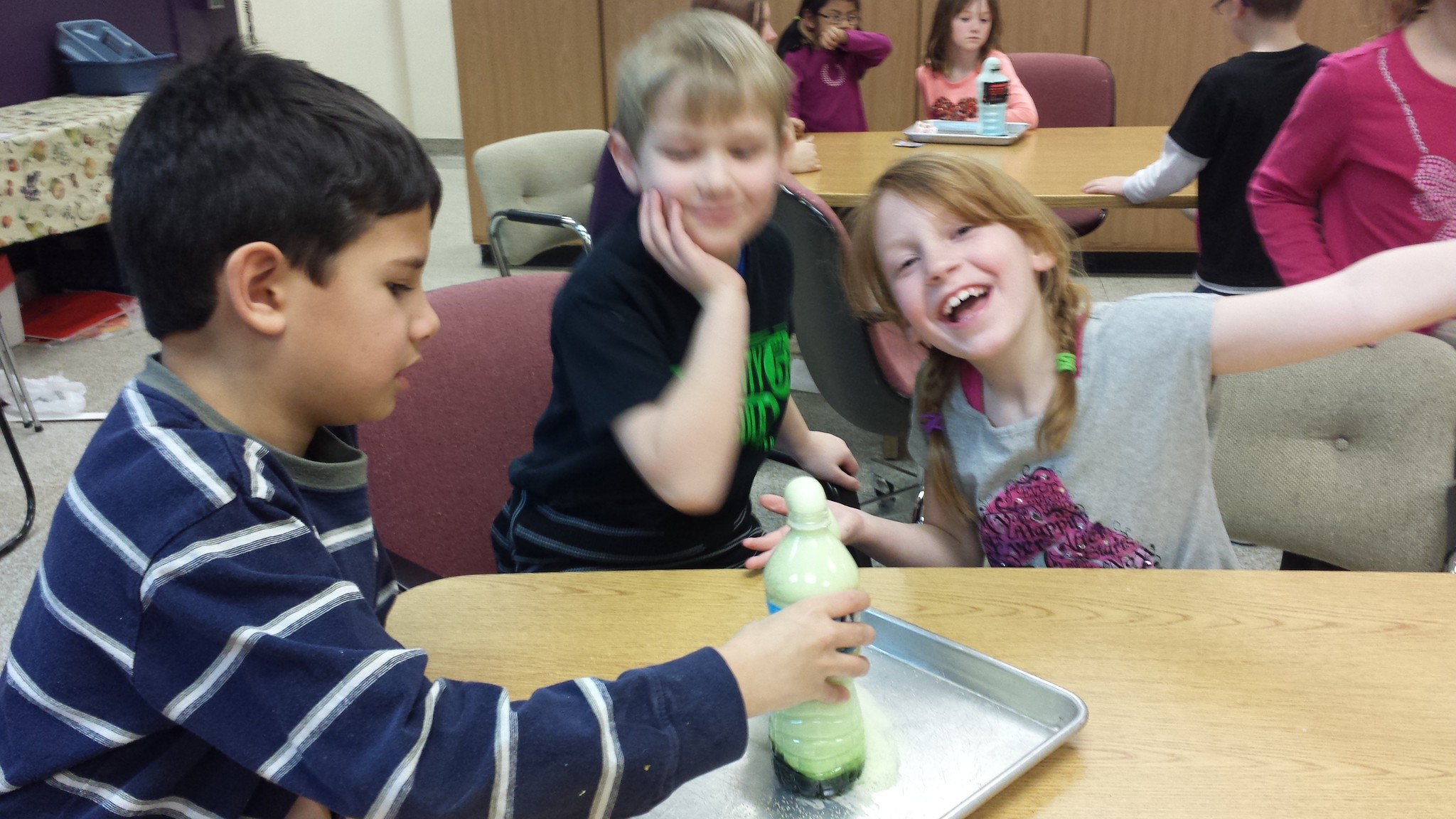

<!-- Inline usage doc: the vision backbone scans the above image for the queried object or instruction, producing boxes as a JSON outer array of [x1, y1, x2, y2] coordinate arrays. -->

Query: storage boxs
[[53, 18, 177, 94]]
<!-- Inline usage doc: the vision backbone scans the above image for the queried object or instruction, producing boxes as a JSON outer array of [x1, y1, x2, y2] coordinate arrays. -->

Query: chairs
[[472, 128, 613, 276], [765, 168, 933, 461], [352, 272, 575, 573], [1001, 52, 1116, 240], [1206, 331, 1456, 572]]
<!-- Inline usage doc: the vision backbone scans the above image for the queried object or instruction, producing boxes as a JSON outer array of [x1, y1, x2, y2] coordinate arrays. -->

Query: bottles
[[763, 505, 868, 796], [975, 59, 1009, 136]]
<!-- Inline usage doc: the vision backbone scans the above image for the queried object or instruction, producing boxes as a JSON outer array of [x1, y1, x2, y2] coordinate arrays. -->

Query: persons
[[774, 2, 893, 138], [1243, 1, 1454, 575], [692, 2, 778, 47], [0, 50, 877, 819], [740, 154, 1455, 574], [1081, 0, 1337, 298], [488, 4, 882, 577], [914, 0, 1040, 134]]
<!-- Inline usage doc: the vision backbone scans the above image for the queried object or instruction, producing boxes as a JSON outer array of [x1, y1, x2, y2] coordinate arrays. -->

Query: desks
[[381, 567, 1456, 819], [0, 94, 156, 249], [787, 125, 1199, 209]]
